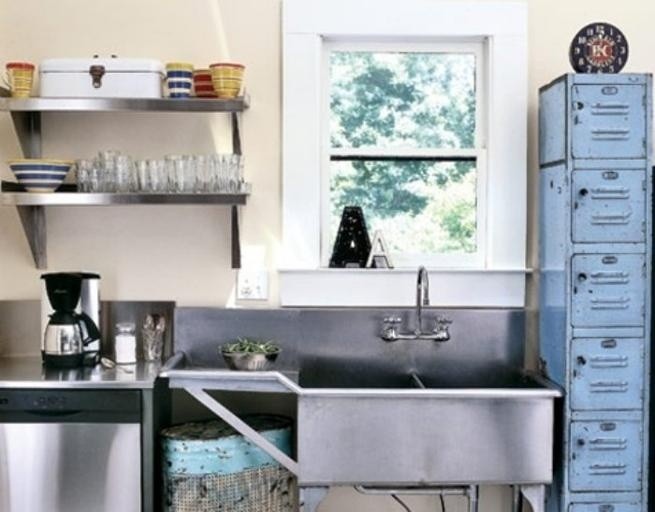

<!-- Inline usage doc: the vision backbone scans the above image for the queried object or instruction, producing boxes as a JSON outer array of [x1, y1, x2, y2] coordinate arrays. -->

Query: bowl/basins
[[6, 158, 74, 193], [219, 347, 282, 370]]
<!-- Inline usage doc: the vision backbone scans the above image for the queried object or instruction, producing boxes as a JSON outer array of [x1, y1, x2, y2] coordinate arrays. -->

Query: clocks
[[569, 23, 629, 74]]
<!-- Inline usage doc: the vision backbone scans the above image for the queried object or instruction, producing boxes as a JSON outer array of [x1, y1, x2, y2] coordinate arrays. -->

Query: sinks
[[413, 372, 566, 486], [297, 370, 425, 487]]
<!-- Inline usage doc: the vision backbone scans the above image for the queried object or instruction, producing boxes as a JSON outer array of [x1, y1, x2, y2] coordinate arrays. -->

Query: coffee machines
[[39, 270, 103, 368]]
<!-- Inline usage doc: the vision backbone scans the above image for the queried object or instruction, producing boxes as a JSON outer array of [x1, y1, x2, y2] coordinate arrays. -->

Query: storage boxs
[[37, 57, 167, 99]]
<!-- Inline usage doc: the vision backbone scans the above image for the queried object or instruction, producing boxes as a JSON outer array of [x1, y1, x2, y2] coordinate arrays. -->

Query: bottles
[[115, 322, 136, 364]]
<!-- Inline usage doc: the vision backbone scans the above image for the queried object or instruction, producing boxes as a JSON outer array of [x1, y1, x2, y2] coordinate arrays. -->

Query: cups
[[164, 62, 245, 99], [142, 326, 163, 363], [76, 151, 246, 196], [2, 62, 35, 96]]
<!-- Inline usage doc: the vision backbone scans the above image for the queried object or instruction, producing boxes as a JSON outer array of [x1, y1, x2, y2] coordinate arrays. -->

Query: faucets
[[417, 267, 429, 332]]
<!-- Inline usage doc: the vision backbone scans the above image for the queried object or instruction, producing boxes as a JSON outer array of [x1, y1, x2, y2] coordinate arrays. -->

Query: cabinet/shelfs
[[539, 73, 653, 168], [539, 158, 653, 512], [0, 87, 252, 270]]
